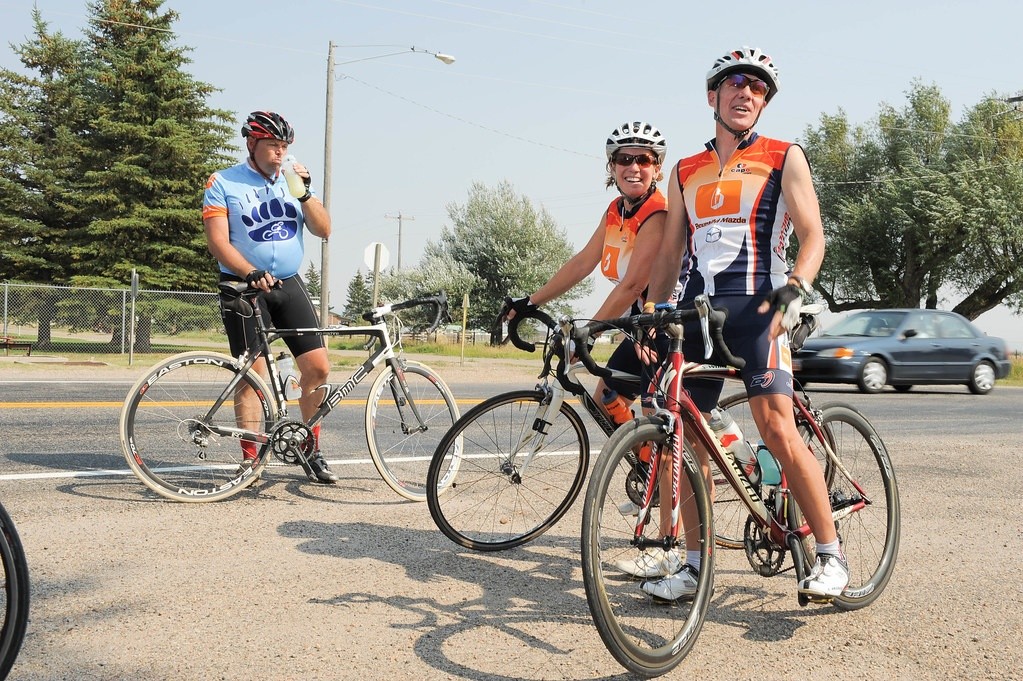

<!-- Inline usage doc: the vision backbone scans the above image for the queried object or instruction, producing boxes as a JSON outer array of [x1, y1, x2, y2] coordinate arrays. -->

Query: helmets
[[241, 111, 294, 144], [707, 46, 781, 105], [606, 122, 668, 166]]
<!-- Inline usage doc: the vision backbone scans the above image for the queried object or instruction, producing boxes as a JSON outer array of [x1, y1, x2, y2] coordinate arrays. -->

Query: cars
[[790, 308, 1013, 394]]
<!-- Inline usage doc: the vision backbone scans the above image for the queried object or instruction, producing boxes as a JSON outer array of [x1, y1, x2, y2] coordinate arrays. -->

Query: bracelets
[[789, 274, 816, 297]]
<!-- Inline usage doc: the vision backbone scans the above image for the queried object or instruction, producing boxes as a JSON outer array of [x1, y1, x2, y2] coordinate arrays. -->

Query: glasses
[[725, 74, 770, 96], [613, 153, 657, 167]]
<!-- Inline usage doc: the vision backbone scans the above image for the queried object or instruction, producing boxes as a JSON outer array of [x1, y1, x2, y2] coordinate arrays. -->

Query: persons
[[633, 48, 850, 602], [499, 120, 688, 578], [203, 112, 338, 487]]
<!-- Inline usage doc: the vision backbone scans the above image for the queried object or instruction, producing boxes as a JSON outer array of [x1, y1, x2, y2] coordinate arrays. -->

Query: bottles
[[756, 437, 782, 485], [639, 440, 653, 463], [709, 408, 760, 485], [601, 387, 634, 425], [275, 351, 302, 401], [282, 154, 306, 199]]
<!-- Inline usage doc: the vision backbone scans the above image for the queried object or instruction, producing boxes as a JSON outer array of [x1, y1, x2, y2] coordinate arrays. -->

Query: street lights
[[319, 39, 457, 348]]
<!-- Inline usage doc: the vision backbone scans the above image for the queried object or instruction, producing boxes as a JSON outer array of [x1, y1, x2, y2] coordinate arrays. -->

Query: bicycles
[[425, 295, 901, 677], [118, 280, 463, 503]]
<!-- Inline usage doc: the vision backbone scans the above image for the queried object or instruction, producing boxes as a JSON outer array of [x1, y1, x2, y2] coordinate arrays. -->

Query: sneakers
[[618, 490, 660, 515], [292, 439, 340, 482], [639, 563, 715, 603], [237, 458, 262, 482], [614, 546, 682, 578], [798, 552, 851, 596]]
